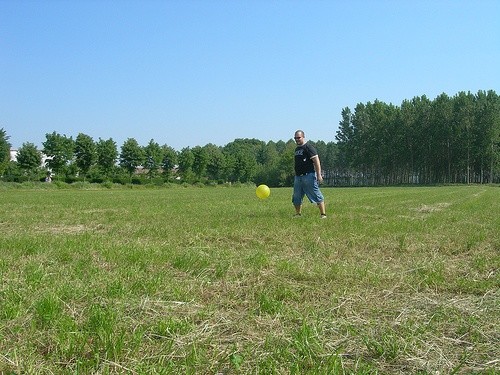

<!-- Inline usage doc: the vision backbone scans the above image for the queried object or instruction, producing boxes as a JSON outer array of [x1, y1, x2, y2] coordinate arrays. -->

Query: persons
[[290, 129, 326, 217]]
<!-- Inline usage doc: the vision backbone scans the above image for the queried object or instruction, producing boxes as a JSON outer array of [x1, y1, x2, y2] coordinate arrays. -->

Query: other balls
[[255, 184, 271, 200]]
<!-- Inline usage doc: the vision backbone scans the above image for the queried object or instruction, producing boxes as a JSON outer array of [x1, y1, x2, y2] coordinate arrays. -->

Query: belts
[[296, 172, 309, 177]]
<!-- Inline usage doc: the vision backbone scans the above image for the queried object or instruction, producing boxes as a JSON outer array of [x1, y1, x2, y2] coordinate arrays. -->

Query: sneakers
[[320, 214, 326, 219], [291, 214, 301, 218]]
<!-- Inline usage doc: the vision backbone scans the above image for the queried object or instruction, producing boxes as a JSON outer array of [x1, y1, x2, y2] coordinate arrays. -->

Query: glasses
[[293, 137, 301, 139]]
